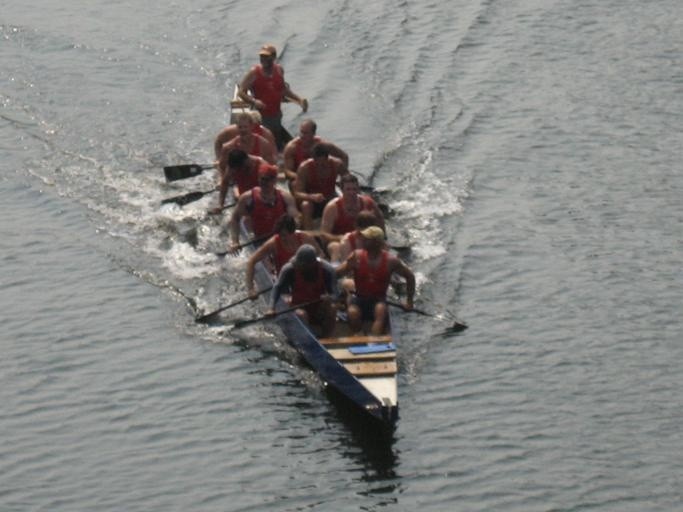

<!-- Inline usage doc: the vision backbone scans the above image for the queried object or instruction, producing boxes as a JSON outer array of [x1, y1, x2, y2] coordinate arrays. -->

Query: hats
[[257, 44, 276, 55], [361, 226, 384, 240], [259, 165, 278, 177], [289, 244, 316, 267], [308, 144, 329, 155]]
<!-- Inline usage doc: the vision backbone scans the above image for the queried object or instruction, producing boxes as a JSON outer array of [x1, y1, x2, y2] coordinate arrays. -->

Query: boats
[[221, 83, 401, 427]]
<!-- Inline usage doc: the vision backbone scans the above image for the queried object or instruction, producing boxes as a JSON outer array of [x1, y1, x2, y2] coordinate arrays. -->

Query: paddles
[[377, 202, 393, 218], [164, 164, 215, 180], [350, 289, 468, 330], [235, 297, 319, 327], [162, 188, 217, 205], [194, 286, 272, 321], [390, 245, 407, 253], [334, 180, 373, 193]]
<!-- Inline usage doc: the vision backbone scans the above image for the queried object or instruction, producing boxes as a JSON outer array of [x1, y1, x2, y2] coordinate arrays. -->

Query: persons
[[215, 110, 415, 334], [238, 46, 308, 148]]
